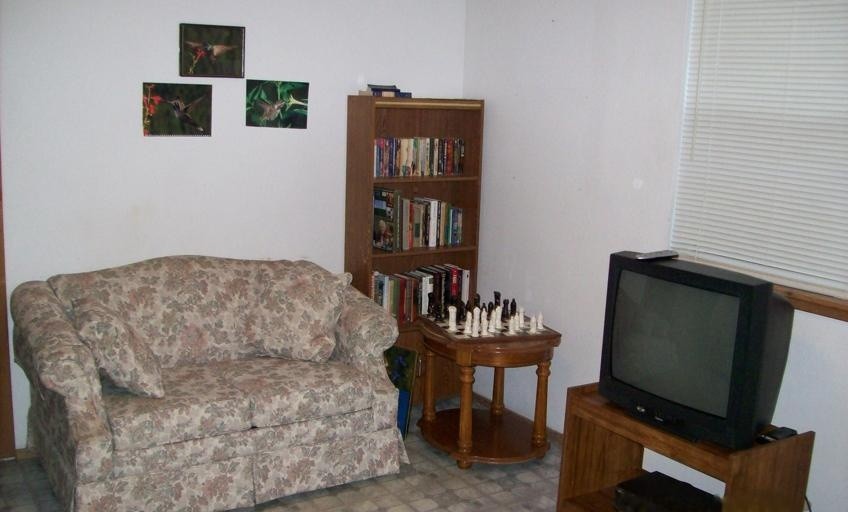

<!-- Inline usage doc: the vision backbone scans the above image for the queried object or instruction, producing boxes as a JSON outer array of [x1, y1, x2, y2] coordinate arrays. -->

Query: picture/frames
[[180, 22, 245, 78]]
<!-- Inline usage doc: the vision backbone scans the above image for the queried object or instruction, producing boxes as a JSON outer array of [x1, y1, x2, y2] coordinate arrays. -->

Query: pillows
[[71, 268, 352, 398]]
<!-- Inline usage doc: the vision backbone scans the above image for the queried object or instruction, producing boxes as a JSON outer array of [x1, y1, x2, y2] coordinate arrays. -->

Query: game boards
[[421, 308, 557, 340]]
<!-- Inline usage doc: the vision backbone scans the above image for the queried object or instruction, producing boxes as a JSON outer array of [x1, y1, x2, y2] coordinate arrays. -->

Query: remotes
[[634, 249, 679, 263]]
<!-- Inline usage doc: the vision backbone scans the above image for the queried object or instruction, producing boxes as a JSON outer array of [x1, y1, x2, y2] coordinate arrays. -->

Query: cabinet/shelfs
[[555, 381, 814, 512], [344, 94, 485, 405]]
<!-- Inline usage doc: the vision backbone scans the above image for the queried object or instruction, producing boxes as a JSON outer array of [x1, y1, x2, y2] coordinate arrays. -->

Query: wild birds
[[253, 98, 286, 122], [165, 94, 207, 134], [185, 37, 238, 60]]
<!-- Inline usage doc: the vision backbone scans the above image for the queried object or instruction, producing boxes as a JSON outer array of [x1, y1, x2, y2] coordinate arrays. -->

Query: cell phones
[[759, 426, 796, 444]]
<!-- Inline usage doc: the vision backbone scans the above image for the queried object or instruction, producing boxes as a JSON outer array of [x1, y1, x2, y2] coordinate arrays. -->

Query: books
[[373, 134, 473, 326], [367, 83, 399, 91], [358, 90, 412, 99]]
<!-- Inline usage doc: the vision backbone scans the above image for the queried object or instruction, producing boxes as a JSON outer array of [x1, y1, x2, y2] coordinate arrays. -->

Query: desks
[[420, 318, 561, 468]]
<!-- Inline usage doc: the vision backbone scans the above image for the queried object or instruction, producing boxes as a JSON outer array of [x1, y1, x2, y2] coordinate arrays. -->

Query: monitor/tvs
[[597, 250, 794, 453]]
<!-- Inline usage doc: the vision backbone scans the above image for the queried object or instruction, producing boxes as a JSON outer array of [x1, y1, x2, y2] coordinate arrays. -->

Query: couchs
[[10, 256, 412, 512]]
